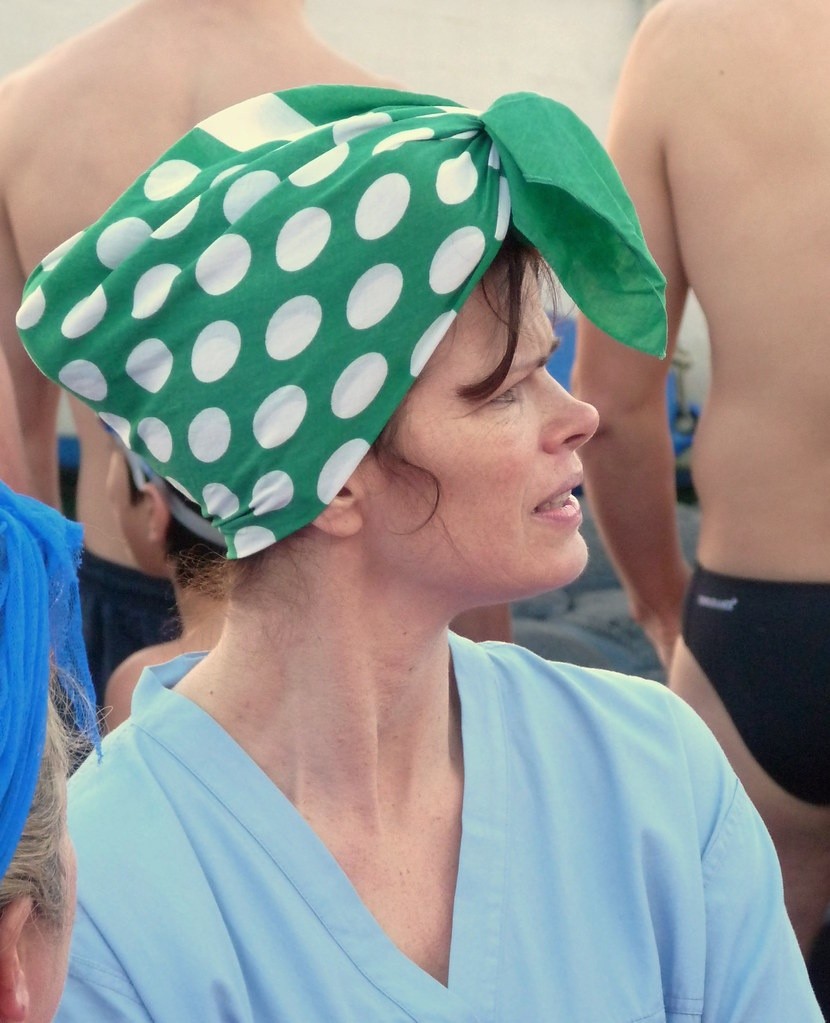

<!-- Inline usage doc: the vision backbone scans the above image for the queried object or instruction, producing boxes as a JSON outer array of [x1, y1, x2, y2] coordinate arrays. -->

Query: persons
[[49, 86, 824, 1023], [568, 0, 830, 964], [0, 478, 111, 1023], [0, 0, 508, 736]]
